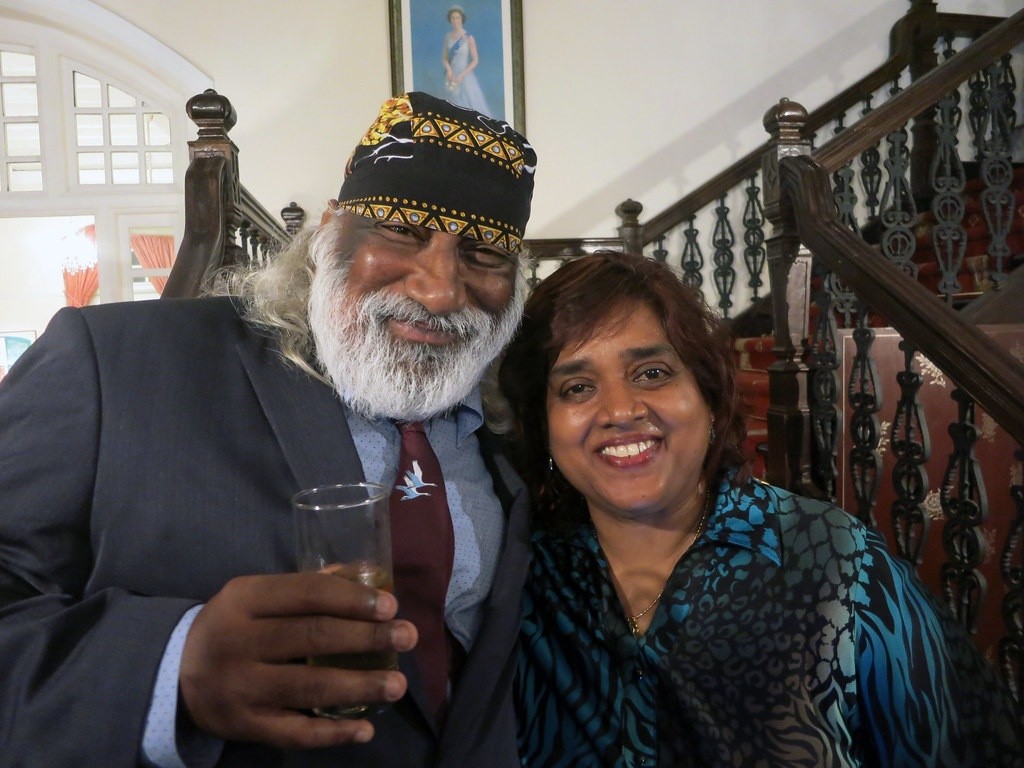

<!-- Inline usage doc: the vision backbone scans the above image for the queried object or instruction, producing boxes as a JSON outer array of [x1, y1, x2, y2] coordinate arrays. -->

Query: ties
[[388, 420, 455, 740]]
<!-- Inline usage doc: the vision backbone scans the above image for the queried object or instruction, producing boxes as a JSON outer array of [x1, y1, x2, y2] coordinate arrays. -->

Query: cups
[[288, 482, 401, 721]]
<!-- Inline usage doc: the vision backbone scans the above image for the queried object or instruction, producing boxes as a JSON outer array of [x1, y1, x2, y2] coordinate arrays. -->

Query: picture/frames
[[389, 1, 526, 141]]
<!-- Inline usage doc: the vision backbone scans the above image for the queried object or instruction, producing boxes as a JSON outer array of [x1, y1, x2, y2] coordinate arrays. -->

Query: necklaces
[[626, 490, 710, 635]]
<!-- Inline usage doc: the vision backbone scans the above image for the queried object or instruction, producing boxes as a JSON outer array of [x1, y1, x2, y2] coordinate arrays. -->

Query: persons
[[439, 7, 488, 112], [499, 250, 1024, 768], [0, 91, 537, 768]]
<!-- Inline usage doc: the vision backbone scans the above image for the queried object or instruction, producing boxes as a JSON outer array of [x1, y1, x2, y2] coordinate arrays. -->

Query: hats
[[338, 92, 538, 255]]
